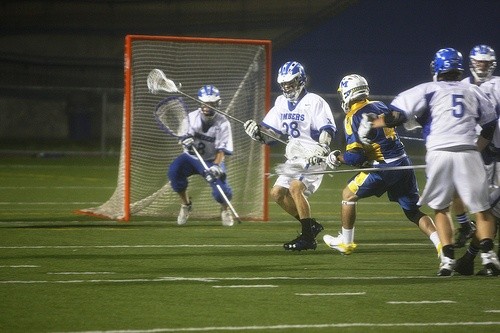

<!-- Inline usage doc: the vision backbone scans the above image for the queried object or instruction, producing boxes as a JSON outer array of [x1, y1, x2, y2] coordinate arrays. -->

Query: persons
[[323, 74, 442, 258], [167, 85, 235, 226], [244, 61, 336, 250], [357, 49, 500, 277], [455, 45, 500, 248]]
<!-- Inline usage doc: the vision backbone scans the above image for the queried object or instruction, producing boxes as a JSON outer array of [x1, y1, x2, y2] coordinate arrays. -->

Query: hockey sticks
[[152, 95, 242, 224], [274, 163, 426, 177], [146, 68, 288, 146], [286, 139, 346, 164]]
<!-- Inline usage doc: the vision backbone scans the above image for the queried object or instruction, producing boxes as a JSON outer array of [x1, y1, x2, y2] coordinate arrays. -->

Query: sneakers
[[453, 256, 474, 275], [221, 207, 234, 226], [475, 255, 500, 276], [481, 249, 500, 276], [177, 201, 192, 225], [454, 221, 477, 248], [322, 231, 357, 256], [283, 236, 317, 252], [300, 222, 324, 239], [437, 256, 455, 276]]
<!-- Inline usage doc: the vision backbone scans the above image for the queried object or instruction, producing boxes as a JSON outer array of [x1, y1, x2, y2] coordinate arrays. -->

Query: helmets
[[429, 48, 464, 82], [337, 74, 369, 115], [276, 61, 307, 102], [469, 45, 497, 83], [198, 85, 222, 120]]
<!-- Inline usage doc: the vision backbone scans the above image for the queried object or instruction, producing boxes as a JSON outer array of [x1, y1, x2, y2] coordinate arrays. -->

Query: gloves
[[203, 165, 225, 183], [243, 120, 265, 144], [326, 150, 341, 170], [304, 143, 331, 166], [357, 113, 376, 146], [179, 134, 195, 150]]
